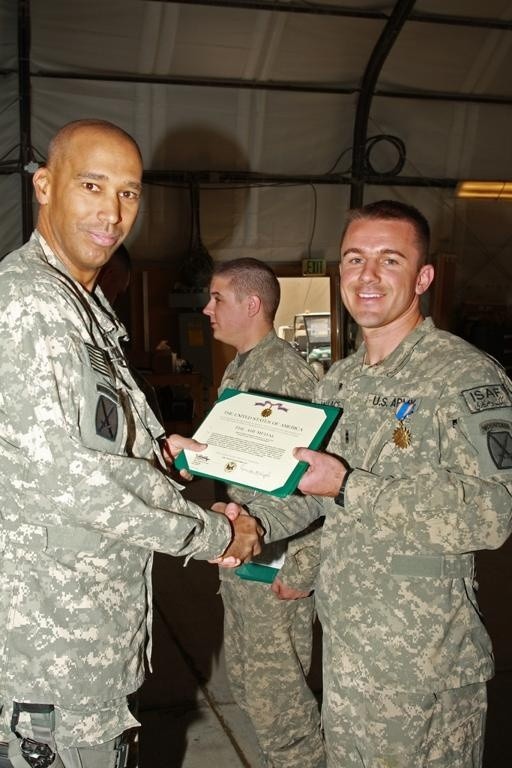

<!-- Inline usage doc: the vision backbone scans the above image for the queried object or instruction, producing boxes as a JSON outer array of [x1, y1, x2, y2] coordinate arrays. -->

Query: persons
[[205, 196, 511, 767], [195, 255, 328, 768], [0, 116, 268, 768]]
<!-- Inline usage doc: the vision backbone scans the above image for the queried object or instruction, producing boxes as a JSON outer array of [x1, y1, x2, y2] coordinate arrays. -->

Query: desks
[[131, 369, 204, 437]]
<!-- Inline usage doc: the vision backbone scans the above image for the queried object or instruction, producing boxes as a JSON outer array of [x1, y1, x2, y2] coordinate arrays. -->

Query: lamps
[[453, 180, 511, 200]]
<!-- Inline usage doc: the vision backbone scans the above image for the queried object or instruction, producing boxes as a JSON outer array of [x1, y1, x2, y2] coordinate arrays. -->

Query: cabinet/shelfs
[[293, 312, 332, 364]]
